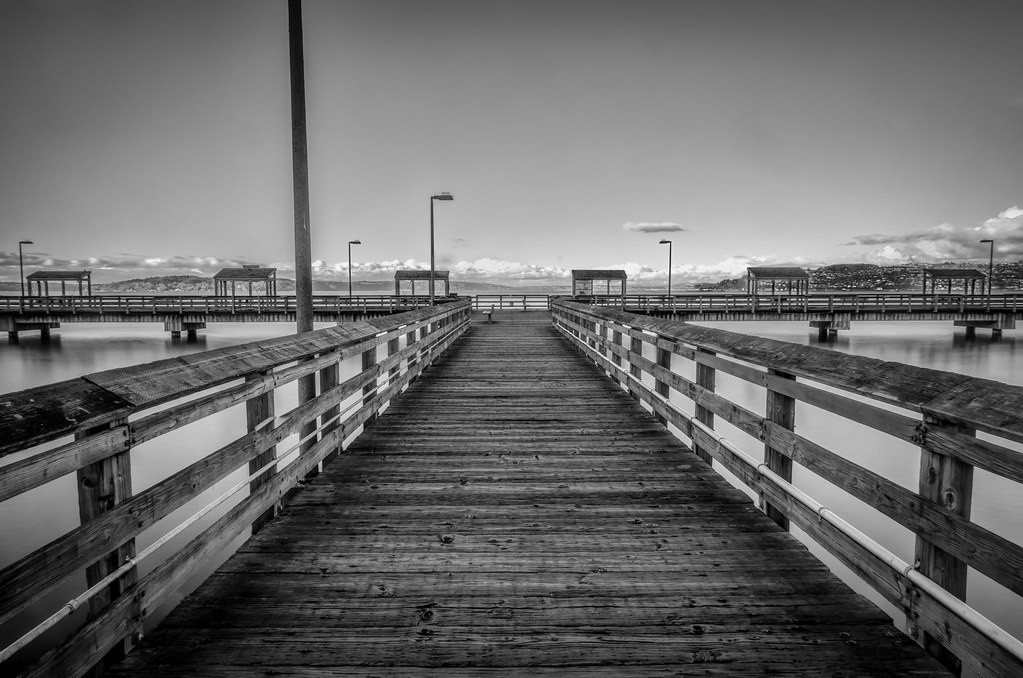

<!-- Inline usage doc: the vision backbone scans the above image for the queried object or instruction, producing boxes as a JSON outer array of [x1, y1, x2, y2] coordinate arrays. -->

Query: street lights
[[19, 241, 33, 311], [348, 240, 361, 308], [659, 240, 672, 308], [429, 194, 454, 307], [980, 239, 995, 312]]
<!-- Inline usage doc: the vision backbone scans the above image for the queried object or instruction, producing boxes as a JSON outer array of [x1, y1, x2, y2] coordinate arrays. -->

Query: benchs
[[483, 304, 494, 324]]
